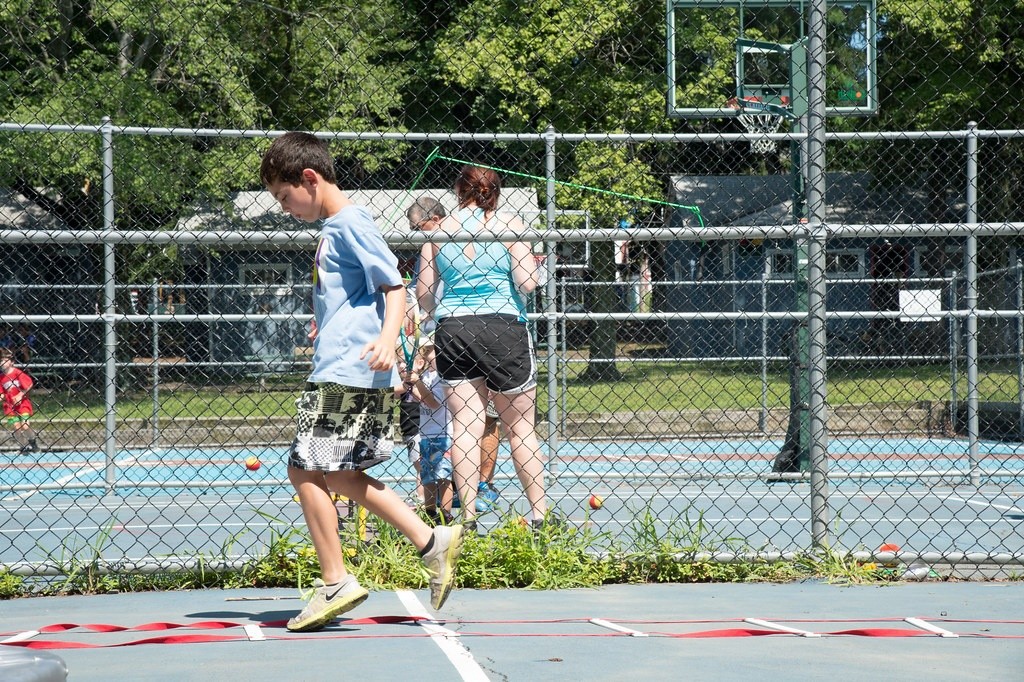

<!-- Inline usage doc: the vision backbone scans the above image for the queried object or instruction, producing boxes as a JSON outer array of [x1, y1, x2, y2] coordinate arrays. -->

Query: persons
[[416, 162, 568, 543], [259, 132, 465, 632], [402, 329, 454, 529], [0, 349, 40, 457], [406, 197, 502, 514], [0, 324, 39, 368], [394, 341, 431, 507]]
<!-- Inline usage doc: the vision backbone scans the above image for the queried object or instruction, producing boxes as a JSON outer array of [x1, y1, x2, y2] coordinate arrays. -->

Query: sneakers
[[417, 525, 464, 610], [19, 447, 29, 456], [29, 439, 41, 453], [439, 511, 457, 525], [403, 495, 427, 511], [436, 493, 462, 508], [286, 576, 370, 632], [474, 484, 501, 512], [461, 519, 479, 540], [532, 516, 570, 541]]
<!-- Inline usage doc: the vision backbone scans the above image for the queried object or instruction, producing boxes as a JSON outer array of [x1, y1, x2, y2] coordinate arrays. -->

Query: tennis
[[587, 495, 603, 508], [245, 456, 260, 470]]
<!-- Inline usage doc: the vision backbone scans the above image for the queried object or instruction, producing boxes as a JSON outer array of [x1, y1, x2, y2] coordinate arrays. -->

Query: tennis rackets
[[400, 287, 422, 382]]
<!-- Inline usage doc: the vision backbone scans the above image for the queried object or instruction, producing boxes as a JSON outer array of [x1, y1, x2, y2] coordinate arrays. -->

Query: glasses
[[410, 215, 431, 232]]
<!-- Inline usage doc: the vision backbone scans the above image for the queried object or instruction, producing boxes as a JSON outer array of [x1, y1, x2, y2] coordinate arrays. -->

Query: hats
[[418, 331, 435, 346]]
